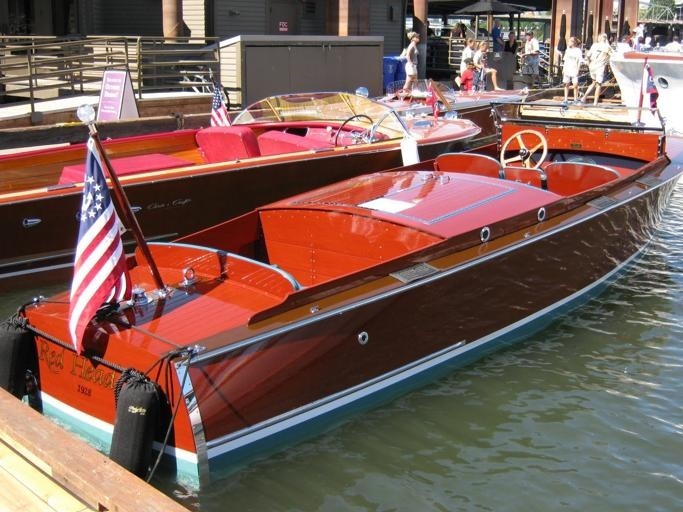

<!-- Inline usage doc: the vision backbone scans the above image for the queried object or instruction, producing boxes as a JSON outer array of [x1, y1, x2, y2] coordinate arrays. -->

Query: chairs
[[258, 131, 326, 155], [432, 153, 504, 182], [195, 127, 258, 161], [542, 160, 620, 196]]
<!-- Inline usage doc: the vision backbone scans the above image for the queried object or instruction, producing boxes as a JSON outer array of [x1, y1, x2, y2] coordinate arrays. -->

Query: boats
[[0, 87, 487, 294], [606, 49, 682, 137], [0, 57, 682, 500], [316, 85, 534, 157]]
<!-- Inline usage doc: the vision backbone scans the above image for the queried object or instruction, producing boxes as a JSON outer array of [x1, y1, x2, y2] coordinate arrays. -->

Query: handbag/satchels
[[400, 49, 410, 62]]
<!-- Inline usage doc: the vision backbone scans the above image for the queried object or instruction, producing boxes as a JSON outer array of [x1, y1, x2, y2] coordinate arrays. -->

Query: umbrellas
[[453, 1, 521, 39], [557, 11, 566, 73]]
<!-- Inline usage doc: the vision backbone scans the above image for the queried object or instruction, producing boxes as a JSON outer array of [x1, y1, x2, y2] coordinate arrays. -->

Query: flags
[[642, 62, 657, 115], [66, 134, 132, 359], [209, 86, 231, 127]]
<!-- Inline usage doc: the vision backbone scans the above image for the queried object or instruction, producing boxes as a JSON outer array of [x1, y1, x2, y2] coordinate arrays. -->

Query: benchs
[[130, 239, 298, 300]]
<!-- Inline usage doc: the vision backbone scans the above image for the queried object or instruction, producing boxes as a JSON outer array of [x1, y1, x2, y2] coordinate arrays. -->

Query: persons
[[459, 37, 476, 76], [397, 31, 421, 93], [491, 19, 504, 51], [580, 32, 614, 106], [472, 40, 504, 91], [521, 30, 540, 86], [562, 37, 583, 104], [607, 19, 682, 55], [503, 32, 519, 72]]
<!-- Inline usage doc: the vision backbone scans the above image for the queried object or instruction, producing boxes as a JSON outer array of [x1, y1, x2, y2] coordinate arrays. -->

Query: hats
[[408, 32, 420, 39], [526, 31, 534, 35]]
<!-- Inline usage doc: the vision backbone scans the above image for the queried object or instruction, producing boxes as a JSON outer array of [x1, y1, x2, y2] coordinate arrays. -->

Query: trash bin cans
[[383, 55, 406, 90]]
